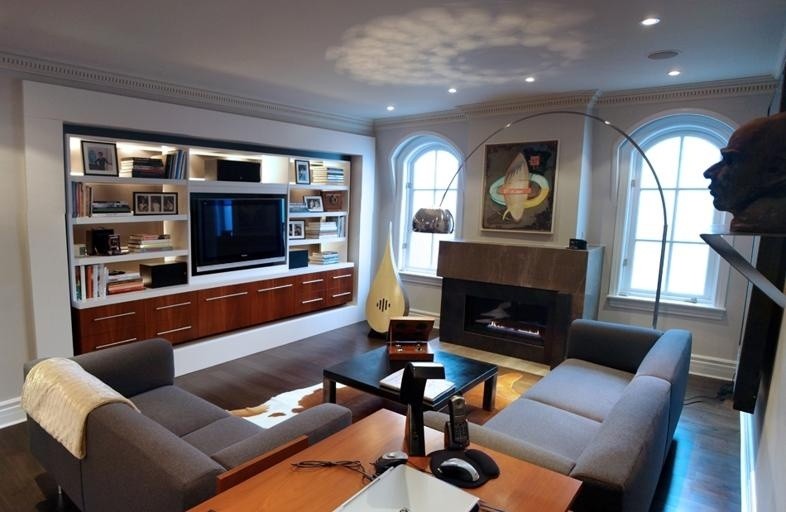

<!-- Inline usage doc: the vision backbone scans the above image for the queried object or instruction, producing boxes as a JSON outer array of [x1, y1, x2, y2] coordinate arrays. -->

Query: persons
[[700, 110, 786, 236]]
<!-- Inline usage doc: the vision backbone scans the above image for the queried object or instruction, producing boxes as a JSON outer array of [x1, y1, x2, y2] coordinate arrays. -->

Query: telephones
[[444, 396, 469, 450]]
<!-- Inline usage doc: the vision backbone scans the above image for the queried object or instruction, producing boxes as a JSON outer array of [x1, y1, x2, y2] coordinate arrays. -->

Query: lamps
[[410, 109, 669, 331]]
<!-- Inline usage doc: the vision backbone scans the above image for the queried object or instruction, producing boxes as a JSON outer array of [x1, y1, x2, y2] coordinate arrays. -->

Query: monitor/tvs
[[190, 193, 287, 276]]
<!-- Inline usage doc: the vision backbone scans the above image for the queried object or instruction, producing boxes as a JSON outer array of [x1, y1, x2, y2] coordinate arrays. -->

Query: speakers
[[140, 261, 187, 287], [207, 159, 260, 182], [289, 247, 308, 269]]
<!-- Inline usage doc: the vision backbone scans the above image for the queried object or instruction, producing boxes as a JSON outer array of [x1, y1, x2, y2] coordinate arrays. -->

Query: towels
[[18, 356, 142, 461]]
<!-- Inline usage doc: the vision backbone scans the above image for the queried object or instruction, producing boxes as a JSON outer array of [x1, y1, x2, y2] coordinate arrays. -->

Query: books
[[379, 385, 457, 407], [379, 365, 456, 402], [71, 148, 185, 301], [288, 160, 344, 268]]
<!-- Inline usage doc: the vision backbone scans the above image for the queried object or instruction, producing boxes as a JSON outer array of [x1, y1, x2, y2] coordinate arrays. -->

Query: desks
[[184, 408, 585, 512]]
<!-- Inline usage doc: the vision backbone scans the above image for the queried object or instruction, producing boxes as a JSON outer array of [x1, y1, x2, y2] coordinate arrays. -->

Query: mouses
[[437, 458, 481, 483]]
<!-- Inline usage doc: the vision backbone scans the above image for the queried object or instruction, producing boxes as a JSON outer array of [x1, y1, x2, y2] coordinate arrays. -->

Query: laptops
[[331, 464, 480, 512]]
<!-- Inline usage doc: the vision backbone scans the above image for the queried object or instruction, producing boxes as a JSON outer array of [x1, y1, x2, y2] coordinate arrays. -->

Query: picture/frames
[[293, 159, 310, 185], [131, 191, 178, 216], [80, 139, 119, 177], [302, 195, 325, 213], [64, 132, 189, 310], [288, 219, 305, 240], [477, 135, 561, 236], [729, 56, 785, 416], [319, 190, 343, 211]]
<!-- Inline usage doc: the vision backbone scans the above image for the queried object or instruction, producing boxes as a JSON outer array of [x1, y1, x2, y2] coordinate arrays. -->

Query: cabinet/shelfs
[[287, 154, 350, 276], [295, 270, 325, 316], [196, 282, 253, 338], [69, 300, 144, 355], [144, 289, 196, 349], [326, 266, 354, 310], [252, 276, 295, 326]]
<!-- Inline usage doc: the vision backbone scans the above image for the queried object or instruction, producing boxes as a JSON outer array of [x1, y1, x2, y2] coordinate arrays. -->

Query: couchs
[[421, 318, 693, 512], [18, 337, 353, 512]]
